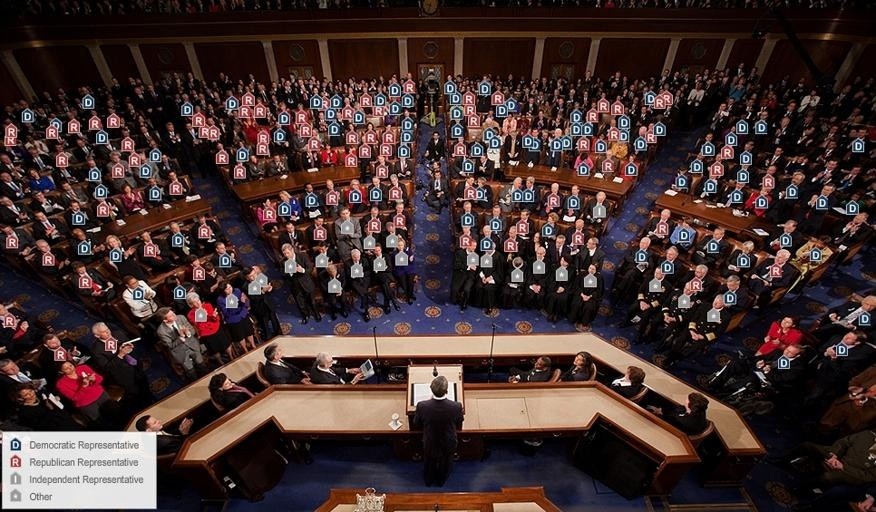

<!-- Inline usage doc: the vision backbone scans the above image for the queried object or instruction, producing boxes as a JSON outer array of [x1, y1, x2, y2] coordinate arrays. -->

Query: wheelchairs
[[702, 349, 776, 419]]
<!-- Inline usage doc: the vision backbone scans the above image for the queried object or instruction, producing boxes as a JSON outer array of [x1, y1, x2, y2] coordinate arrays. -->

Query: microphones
[[433, 360, 439, 376], [368, 326, 376, 331], [492, 323, 503, 329]]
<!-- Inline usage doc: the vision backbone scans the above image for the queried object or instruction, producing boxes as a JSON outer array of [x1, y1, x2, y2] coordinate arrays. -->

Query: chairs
[[256, 91, 417, 303], [689, 420, 715, 450], [254, 362, 273, 388], [209, 393, 227, 415], [646, 137, 876, 397], [546, 369, 563, 383], [443, 71, 611, 328], [1, 105, 254, 428], [629, 387, 649, 404], [587, 362, 597, 381]]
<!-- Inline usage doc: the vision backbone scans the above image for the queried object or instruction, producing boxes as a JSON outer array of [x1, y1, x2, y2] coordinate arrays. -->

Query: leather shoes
[[451, 295, 593, 332], [604, 313, 685, 367]]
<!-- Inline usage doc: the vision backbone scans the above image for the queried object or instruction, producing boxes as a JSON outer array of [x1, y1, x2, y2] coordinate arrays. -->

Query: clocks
[[418, 0, 442, 18]]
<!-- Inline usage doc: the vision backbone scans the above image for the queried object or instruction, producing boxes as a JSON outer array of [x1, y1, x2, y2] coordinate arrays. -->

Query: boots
[[294, 281, 417, 324]]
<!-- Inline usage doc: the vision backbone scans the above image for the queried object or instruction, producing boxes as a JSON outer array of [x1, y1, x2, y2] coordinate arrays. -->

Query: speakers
[[572, 429, 656, 500], [215, 426, 290, 504]]
